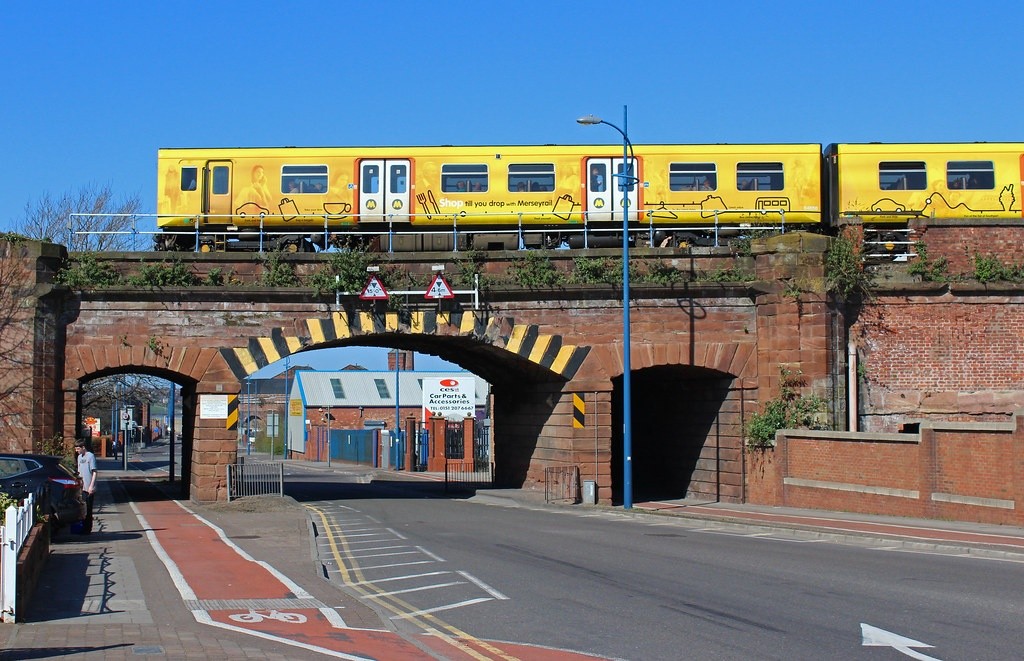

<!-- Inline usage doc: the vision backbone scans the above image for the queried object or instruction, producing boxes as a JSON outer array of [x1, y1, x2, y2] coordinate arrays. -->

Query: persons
[[287, 180, 324, 194], [591, 168, 602, 192], [456, 180, 548, 192], [74, 439, 97, 535]]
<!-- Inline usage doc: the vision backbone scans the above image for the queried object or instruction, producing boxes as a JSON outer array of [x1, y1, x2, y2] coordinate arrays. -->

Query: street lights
[[576, 104, 635, 512]]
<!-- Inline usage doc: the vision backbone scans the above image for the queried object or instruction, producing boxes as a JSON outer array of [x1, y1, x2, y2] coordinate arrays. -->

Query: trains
[[159, 135, 1024, 253]]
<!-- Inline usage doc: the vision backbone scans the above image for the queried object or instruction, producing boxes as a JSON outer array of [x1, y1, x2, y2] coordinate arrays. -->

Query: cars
[[0, 451, 88, 537]]
